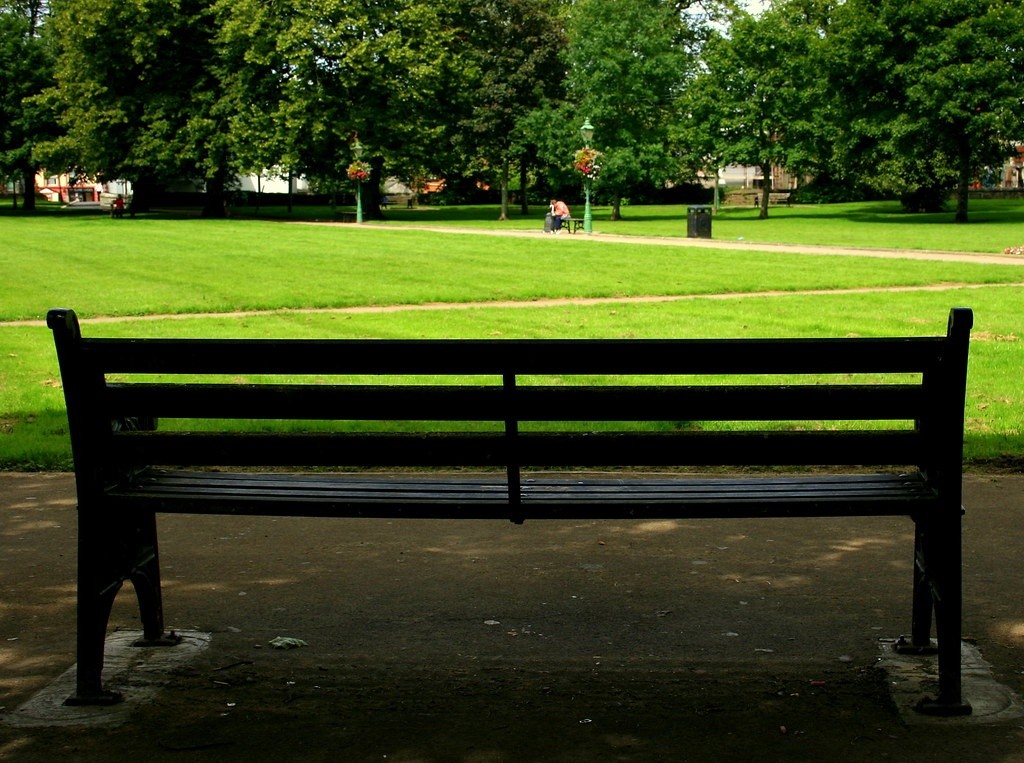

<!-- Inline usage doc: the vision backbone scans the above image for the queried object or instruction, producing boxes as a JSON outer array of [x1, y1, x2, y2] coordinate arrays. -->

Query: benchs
[[46, 308, 977, 720], [110, 202, 151, 219], [757, 193, 793, 209], [553, 219, 584, 234]]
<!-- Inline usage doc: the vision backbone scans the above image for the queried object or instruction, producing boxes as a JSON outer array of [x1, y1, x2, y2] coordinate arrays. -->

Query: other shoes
[[556, 229, 561, 234], [551, 229, 556, 234]]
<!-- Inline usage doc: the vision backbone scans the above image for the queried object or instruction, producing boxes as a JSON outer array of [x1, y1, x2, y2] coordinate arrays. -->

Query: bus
[[38, 185, 98, 205]]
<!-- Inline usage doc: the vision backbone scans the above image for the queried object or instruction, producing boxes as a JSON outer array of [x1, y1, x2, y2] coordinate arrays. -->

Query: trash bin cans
[[687, 205, 712, 238]]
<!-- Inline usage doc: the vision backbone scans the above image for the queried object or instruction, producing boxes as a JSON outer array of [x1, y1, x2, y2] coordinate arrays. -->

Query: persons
[[407, 187, 413, 209], [381, 195, 388, 209], [550, 200, 569, 234], [111, 194, 123, 219]]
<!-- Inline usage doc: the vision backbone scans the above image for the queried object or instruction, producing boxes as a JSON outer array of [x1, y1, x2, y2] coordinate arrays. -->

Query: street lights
[[579, 116, 594, 234]]
